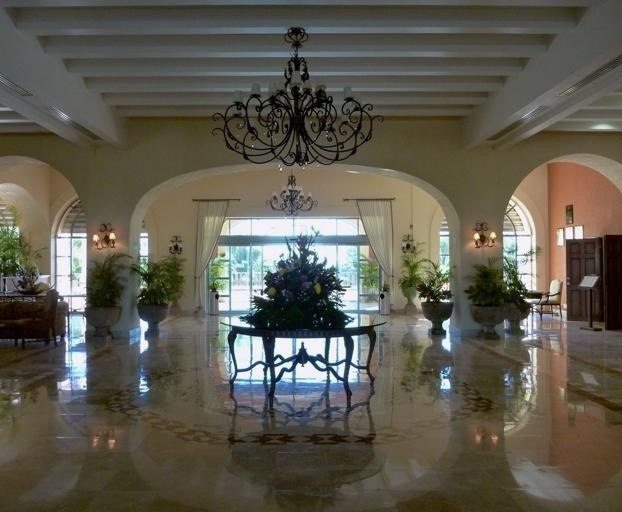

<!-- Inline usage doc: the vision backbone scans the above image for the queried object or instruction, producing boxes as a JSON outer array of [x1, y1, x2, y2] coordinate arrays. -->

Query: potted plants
[[418, 337, 453, 404], [497, 252, 533, 335], [462, 256, 506, 339], [394, 233, 438, 316], [84, 251, 133, 345], [159, 255, 184, 315], [416, 257, 458, 336], [206, 261, 226, 292], [139, 339, 184, 392], [397, 327, 426, 394], [127, 252, 187, 337]]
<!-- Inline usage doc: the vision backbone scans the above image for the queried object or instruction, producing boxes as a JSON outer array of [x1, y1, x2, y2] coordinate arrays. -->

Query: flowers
[[238, 225, 355, 331], [229, 443, 375, 511]]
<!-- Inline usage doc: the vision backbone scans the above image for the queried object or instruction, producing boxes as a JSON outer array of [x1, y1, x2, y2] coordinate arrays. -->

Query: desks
[[522, 292, 550, 321], [221, 311, 388, 417], [222, 423, 385, 488], [0, 292, 47, 303]]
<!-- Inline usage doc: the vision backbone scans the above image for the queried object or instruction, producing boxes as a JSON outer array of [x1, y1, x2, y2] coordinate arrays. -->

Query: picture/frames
[[556, 203, 585, 247]]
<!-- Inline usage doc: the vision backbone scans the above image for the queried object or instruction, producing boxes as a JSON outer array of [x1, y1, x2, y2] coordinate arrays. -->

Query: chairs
[[532, 279, 564, 321]]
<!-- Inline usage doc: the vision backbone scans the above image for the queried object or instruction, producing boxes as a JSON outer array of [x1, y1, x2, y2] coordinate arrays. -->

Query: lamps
[[208, 37, 385, 174], [473, 221, 497, 249], [264, 168, 318, 216], [168, 234, 183, 254], [92, 223, 118, 251]]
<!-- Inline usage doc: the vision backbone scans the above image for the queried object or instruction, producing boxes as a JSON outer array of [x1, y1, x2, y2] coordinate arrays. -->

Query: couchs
[[0, 289, 60, 349]]
